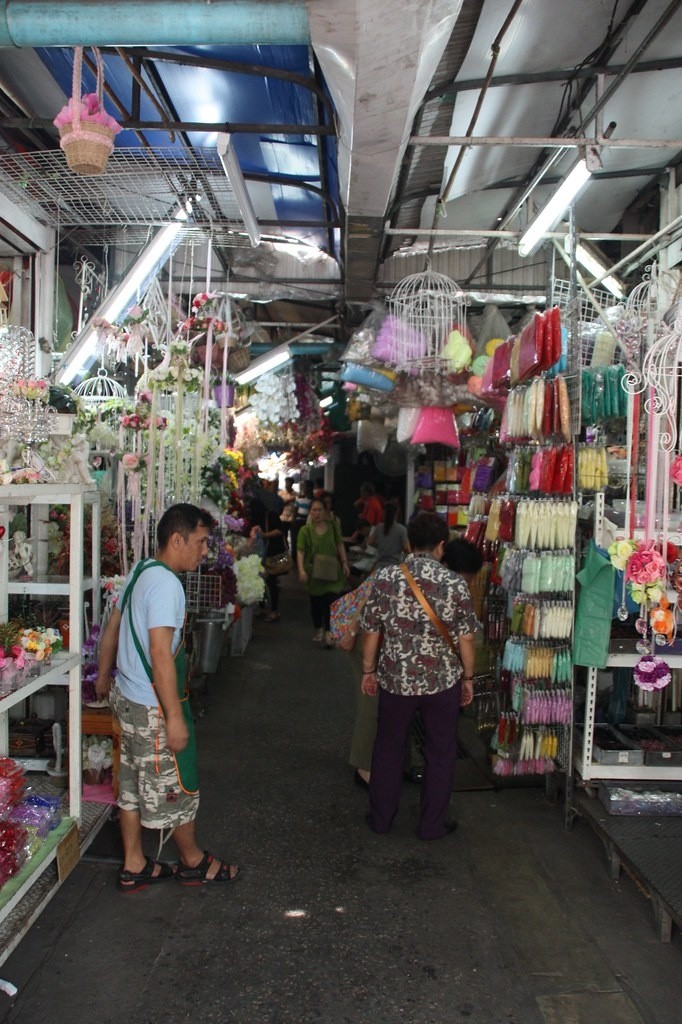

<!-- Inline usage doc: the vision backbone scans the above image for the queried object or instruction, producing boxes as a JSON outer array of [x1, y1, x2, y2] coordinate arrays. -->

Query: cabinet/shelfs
[[422, 278, 682, 831], [0, 481, 141, 966]]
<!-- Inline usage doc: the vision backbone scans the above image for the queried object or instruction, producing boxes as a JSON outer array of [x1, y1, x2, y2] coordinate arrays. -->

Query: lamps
[[518, 147, 603, 258], [563, 222, 628, 302], [217, 131, 261, 247], [56, 185, 204, 388], [235, 347, 294, 386]]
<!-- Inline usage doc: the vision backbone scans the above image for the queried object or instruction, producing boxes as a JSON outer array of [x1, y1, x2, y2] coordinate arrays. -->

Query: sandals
[[116, 856, 178, 894], [175, 850, 240, 885]]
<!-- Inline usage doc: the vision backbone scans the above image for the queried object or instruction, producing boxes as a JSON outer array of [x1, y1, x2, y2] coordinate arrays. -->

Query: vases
[[194, 343, 225, 369], [228, 347, 251, 373], [214, 385, 234, 408], [215, 333, 238, 349]]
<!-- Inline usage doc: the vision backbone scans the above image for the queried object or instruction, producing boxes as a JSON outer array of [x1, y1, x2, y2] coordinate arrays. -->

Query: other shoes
[[326, 638, 335, 647], [355, 768, 369, 790], [255, 607, 265, 616], [264, 611, 280, 622], [313, 636, 324, 643], [448, 820, 457, 833]]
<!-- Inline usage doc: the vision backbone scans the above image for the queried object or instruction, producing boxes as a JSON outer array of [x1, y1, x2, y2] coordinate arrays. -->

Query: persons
[[95, 503, 242, 895], [367, 501, 411, 568], [291, 479, 315, 569], [351, 481, 385, 540], [439, 537, 484, 591], [275, 476, 298, 540], [306, 489, 341, 536], [295, 496, 350, 645], [249, 502, 290, 621], [356, 510, 476, 841]]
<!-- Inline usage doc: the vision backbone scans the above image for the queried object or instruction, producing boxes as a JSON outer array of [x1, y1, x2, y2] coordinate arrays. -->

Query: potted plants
[[35, 387, 78, 437]]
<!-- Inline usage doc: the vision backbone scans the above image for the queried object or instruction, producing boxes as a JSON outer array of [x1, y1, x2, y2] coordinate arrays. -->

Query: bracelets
[[361, 668, 376, 675], [342, 559, 348, 562], [461, 675, 474, 680]]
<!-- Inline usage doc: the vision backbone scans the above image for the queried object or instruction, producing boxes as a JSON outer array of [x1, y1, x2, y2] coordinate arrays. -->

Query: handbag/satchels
[[329, 566, 381, 654], [314, 554, 338, 582], [266, 551, 293, 577]]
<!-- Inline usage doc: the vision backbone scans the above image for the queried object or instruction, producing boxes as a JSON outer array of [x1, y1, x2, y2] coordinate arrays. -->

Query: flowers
[[606, 538, 682, 692], [1, 293, 267, 886], [54, 94, 123, 135]]
